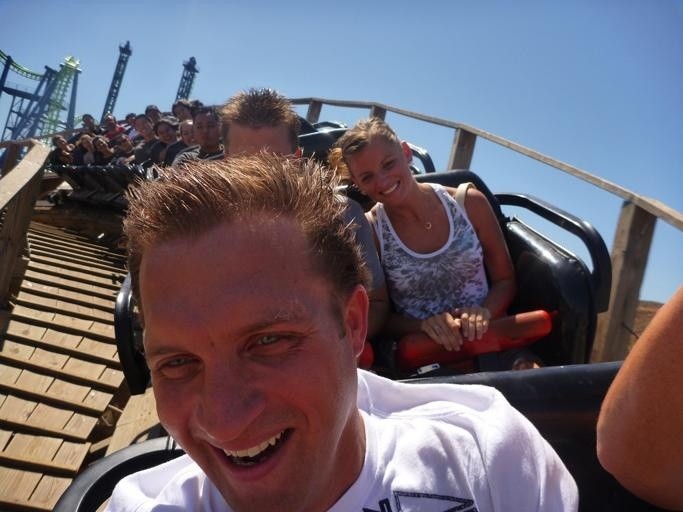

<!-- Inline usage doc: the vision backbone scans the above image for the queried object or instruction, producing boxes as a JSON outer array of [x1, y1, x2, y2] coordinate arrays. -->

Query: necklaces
[[389, 185, 433, 230]]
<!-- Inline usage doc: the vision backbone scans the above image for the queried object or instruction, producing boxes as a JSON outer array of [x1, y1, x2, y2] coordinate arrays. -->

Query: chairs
[[334, 167, 612, 372], [49, 131, 80, 166], [203, 128, 352, 170], [52, 357, 634, 511]]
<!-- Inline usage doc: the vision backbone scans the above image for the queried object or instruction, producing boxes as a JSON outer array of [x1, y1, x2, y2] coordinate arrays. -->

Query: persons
[[132, 113, 159, 161], [53, 135, 78, 165], [81, 135, 97, 164], [218, 87, 388, 338], [124, 113, 141, 139], [101, 150, 580, 512], [146, 105, 162, 124], [172, 107, 225, 167], [174, 119, 200, 160], [92, 136, 120, 165], [104, 113, 126, 139], [113, 133, 136, 163], [339, 118, 540, 380], [81, 112, 103, 135], [172, 97, 192, 120], [596, 284, 681, 511], [155, 120, 179, 162]]
